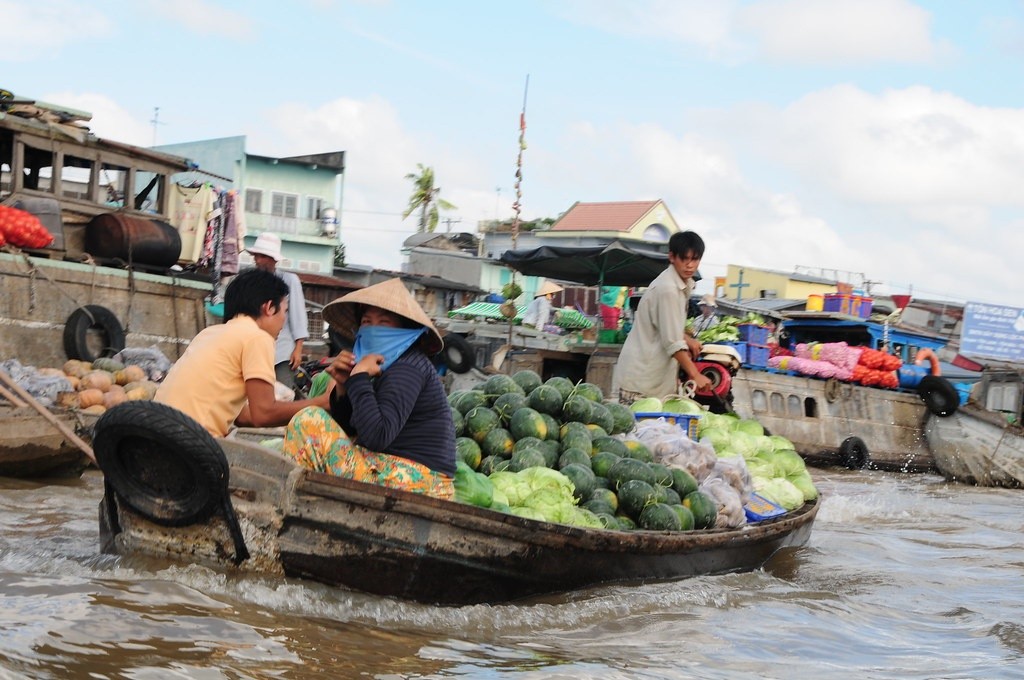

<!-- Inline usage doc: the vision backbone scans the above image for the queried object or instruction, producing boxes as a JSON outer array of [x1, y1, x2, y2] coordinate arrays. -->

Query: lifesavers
[[914, 347, 941, 376]]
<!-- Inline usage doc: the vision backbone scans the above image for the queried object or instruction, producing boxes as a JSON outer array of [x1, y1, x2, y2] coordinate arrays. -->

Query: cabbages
[[630, 394, 817, 508]]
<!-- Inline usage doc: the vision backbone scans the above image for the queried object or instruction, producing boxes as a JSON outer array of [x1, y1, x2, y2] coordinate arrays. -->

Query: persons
[[689, 294, 719, 334], [321, 276, 456, 479], [520, 281, 564, 338], [153, 267, 337, 442], [617, 231, 712, 407], [241, 233, 310, 404]]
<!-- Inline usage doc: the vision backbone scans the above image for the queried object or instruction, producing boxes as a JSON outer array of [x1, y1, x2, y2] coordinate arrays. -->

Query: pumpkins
[[41, 357, 157, 414]]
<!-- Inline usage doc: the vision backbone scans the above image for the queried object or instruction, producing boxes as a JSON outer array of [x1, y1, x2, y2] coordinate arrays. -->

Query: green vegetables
[[686, 312, 766, 344]]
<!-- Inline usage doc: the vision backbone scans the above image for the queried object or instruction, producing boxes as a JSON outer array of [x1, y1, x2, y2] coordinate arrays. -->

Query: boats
[[23, 313, 1024, 595]]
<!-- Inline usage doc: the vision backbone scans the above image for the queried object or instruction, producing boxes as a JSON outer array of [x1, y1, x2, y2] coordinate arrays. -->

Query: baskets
[[741, 492, 787, 523], [584, 327, 627, 344], [636, 413, 700, 444], [717, 325, 771, 363]]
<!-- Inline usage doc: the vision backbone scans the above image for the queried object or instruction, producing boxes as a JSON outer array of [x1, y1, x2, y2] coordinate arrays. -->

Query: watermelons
[[445, 369, 717, 533]]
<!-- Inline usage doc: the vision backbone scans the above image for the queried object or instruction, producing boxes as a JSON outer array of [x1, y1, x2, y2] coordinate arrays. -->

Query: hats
[[697, 295, 718, 308], [245, 233, 285, 262], [532, 281, 565, 299], [322, 276, 444, 357]]
